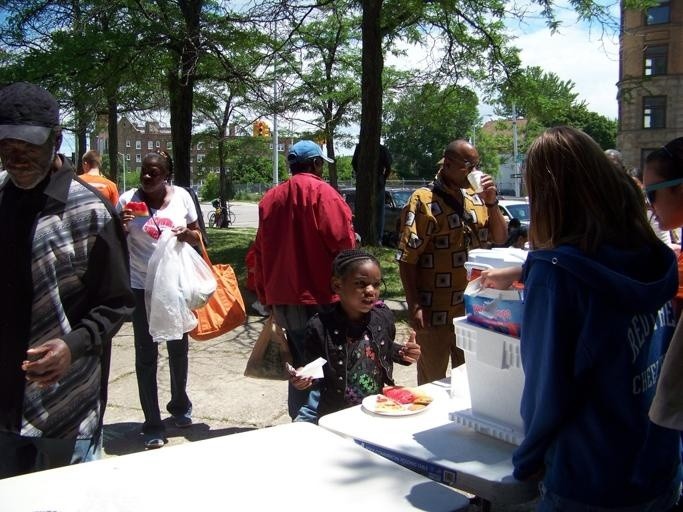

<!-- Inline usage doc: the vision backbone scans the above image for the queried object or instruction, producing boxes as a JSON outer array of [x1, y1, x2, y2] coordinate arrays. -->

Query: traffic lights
[[256, 121, 265, 139], [263, 122, 272, 139]]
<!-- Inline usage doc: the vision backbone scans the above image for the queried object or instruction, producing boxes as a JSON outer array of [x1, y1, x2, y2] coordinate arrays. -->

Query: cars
[[496, 199, 532, 238]]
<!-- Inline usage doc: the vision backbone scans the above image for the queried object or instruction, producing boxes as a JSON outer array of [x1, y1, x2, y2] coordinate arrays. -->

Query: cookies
[[376, 386, 431, 412]]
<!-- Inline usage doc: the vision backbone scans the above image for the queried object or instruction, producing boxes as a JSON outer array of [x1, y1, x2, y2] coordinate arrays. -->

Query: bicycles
[[207, 196, 236, 228]]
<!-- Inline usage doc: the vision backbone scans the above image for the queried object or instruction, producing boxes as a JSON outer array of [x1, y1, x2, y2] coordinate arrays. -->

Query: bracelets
[[484, 199, 498, 209]]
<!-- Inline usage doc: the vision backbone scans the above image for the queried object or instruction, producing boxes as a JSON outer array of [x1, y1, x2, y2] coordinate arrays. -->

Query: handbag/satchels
[[188, 264, 246, 341]]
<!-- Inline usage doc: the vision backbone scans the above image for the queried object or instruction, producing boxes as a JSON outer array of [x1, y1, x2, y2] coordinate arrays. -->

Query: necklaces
[[143, 189, 164, 210]]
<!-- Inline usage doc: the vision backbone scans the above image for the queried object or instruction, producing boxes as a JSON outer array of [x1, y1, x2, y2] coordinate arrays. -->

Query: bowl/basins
[[646, 210, 671, 251]]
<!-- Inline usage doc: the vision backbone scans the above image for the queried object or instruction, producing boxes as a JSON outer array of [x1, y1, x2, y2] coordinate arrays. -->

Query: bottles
[[359, 392, 435, 416]]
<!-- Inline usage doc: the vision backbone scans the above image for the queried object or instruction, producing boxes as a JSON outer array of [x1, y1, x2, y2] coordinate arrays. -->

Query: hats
[[0, 82, 59, 146], [286, 139, 334, 164]]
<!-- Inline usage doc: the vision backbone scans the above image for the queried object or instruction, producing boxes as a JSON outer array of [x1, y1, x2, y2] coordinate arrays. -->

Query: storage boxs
[[453, 247, 525, 449]]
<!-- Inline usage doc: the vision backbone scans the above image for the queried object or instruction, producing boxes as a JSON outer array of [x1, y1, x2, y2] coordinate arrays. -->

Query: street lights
[[483, 81, 521, 199], [117, 151, 126, 193], [473, 114, 492, 147], [263, 15, 278, 188]]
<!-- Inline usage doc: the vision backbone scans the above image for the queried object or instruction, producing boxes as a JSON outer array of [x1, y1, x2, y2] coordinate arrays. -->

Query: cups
[[466, 171, 485, 192]]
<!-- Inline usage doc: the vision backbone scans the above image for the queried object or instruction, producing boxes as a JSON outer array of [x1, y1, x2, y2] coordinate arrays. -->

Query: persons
[[210, 193, 225, 229], [0, 81, 138, 477], [350, 140, 393, 247], [112, 149, 203, 450], [502, 122, 683, 511], [281, 246, 422, 425], [71, 150, 118, 210]]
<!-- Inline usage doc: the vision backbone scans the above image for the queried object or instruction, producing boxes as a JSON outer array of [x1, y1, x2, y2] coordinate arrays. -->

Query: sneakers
[[175, 409, 192, 427], [143, 433, 164, 448]]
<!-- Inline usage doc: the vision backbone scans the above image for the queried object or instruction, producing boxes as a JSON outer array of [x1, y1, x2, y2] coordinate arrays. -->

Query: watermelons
[[122, 202, 150, 218]]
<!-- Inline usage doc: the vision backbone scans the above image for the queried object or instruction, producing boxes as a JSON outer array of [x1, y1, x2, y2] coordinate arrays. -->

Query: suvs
[[334, 186, 422, 248]]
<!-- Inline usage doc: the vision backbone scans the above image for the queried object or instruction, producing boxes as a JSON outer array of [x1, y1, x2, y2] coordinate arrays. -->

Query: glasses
[[642, 178, 683, 203], [448, 155, 480, 168]]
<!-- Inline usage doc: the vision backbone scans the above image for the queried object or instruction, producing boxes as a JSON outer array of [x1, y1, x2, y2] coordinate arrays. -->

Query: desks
[[0, 419, 470, 512], [318, 374, 540, 505]]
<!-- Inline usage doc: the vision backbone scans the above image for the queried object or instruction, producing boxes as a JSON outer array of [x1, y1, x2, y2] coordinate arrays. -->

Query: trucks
[[480, 154, 526, 195]]
